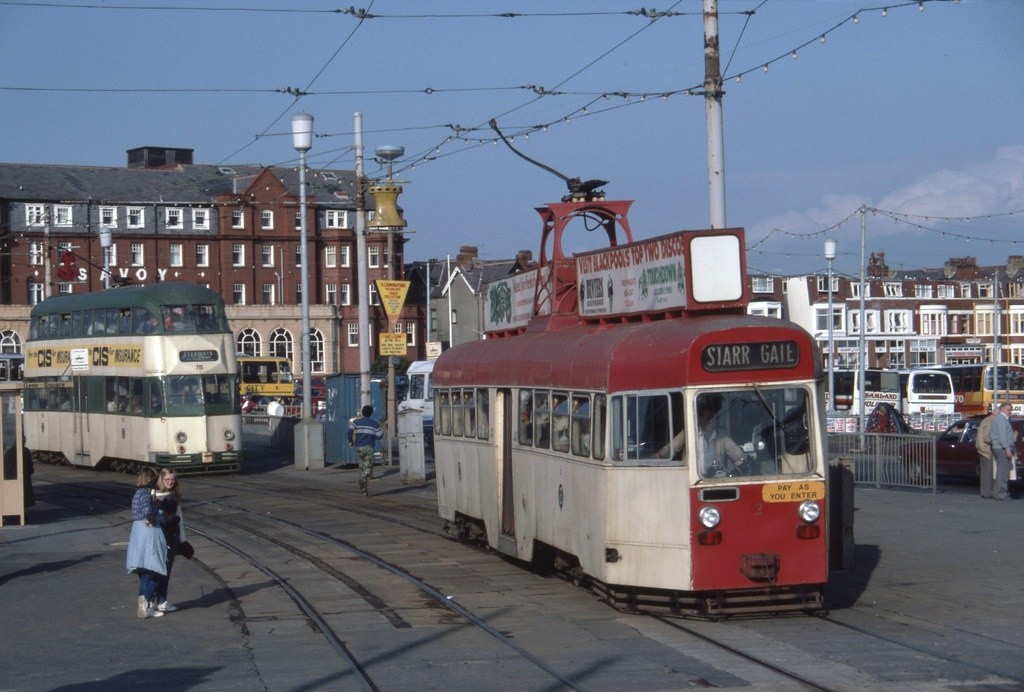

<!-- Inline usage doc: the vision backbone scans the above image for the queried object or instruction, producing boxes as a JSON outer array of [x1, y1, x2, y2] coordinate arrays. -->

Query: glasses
[[163, 478, 175, 482]]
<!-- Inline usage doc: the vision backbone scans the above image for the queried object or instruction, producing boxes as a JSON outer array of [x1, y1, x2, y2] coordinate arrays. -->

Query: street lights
[[290, 112, 325, 470], [824, 239, 836, 412]]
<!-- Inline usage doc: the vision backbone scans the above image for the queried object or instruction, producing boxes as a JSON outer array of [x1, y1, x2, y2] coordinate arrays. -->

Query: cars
[[897, 415, 1024, 485]]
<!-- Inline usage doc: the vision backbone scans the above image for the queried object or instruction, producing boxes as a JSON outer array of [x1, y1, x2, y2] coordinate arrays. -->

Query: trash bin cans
[[829, 463, 854, 568]]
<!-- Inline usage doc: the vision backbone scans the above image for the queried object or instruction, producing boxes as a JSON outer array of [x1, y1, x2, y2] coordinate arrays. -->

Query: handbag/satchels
[[992, 454, 1017, 480], [176, 541, 194, 559]]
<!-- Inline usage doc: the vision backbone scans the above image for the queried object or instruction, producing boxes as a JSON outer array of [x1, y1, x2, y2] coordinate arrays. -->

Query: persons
[[30, 306, 219, 338], [653, 408, 743, 477], [3, 435, 35, 506], [107, 392, 161, 419], [974, 403, 1019, 500], [30, 388, 72, 411], [347, 405, 384, 496], [126, 464, 187, 618]]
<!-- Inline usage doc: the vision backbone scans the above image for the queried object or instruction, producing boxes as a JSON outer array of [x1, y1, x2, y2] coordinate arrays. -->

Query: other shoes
[[358, 479, 369, 496]]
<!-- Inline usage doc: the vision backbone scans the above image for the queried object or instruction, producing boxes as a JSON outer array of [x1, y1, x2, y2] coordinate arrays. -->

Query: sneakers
[[137, 595, 148, 619], [158, 601, 177, 611], [147, 608, 164, 617], [150, 597, 157, 609]]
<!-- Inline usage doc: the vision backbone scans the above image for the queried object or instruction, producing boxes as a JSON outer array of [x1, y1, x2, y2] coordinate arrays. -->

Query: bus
[[916, 363, 1024, 419], [186, 357, 296, 404], [430, 200, 831, 617], [897, 369, 956, 434], [823, 369, 902, 435], [398, 361, 436, 426], [23, 282, 243, 477]]
